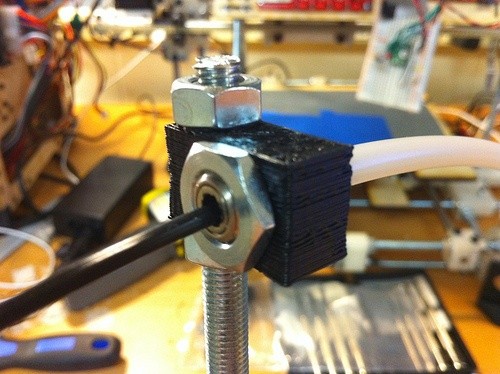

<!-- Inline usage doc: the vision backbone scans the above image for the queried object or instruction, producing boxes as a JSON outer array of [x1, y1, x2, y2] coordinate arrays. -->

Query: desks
[[1, 102, 500, 374]]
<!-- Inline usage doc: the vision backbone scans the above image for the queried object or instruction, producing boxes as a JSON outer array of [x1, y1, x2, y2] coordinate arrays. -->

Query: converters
[[54, 153, 155, 245]]
